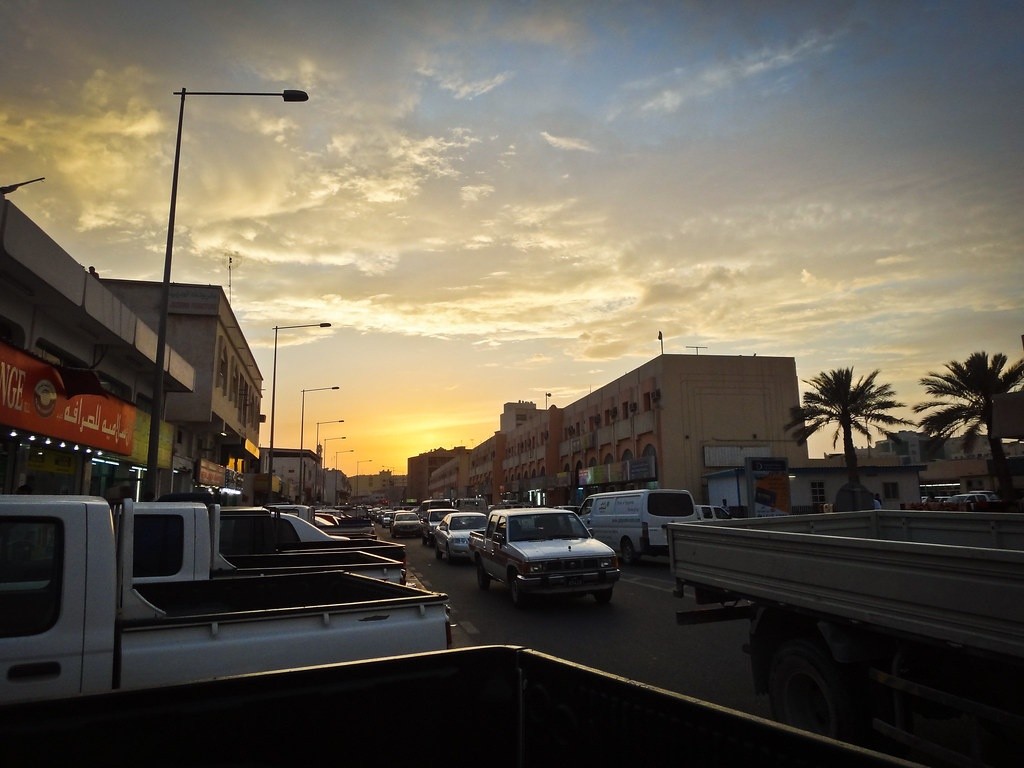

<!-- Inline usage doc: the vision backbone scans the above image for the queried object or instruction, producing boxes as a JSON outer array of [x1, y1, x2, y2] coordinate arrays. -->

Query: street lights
[[298, 386, 340, 505], [335, 449, 353, 504], [266, 322, 331, 504], [356, 459, 371, 506], [316, 420, 343, 504], [324, 436, 346, 504], [146, 88, 308, 506]]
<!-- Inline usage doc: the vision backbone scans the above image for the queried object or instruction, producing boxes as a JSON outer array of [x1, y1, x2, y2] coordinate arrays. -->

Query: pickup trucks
[[0, 503, 407, 587], [469, 510, 621, 608], [420, 509, 458, 545], [1, 646, 928, 767], [267, 506, 377, 538], [212, 508, 406, 569], [1, 494, 453, 767]]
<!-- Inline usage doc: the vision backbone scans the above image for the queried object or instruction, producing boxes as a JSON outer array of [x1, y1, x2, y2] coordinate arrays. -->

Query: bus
[[392, 505, 420, 511]]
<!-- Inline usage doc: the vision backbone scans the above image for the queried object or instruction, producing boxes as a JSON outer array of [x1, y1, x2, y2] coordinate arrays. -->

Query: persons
[[871, 493, 882, 509], [720, 499, 729, 512]]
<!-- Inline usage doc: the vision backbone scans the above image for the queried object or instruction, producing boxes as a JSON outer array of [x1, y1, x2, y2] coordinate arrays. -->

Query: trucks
[[662, 509, 1024, 768]]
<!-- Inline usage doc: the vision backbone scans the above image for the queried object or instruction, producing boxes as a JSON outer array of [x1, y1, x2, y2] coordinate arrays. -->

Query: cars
[[433, 511, 488, 563], [368, 508, 392, 528], [920, 490, 1001, 505], [270, 513, 352, 542], [336, 506, 367, 519], [694, 505, 738, 521], [553, 505, 590, 531], [389, 512, 423, 539]]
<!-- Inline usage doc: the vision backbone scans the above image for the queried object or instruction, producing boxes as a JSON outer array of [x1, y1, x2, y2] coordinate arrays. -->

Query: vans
[[574, 489, 697, 566], [417, 500, 453, 521], [486, 504, 532, 521], [452, 499, 486, 512]]
